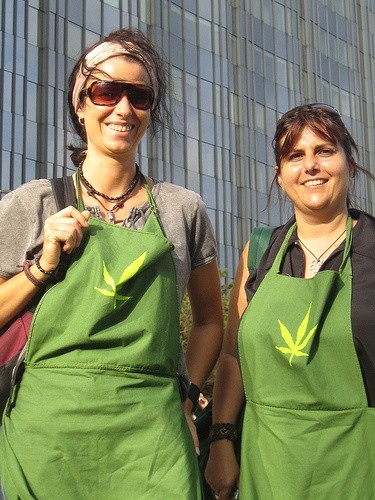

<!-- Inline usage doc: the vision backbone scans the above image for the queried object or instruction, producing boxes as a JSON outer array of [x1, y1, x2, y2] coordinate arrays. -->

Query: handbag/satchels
[[193, 398, 245, 500], [0, 298, 41, 415]]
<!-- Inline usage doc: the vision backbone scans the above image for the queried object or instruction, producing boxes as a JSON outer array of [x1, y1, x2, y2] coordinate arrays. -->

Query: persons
[[0, 28, 226, 500], [205, 103, 375, 500]]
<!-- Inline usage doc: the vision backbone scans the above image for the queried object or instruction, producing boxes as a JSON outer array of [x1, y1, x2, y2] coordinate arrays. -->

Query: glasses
[[80, 80, 156, 111], [280, 102, 342, 123]]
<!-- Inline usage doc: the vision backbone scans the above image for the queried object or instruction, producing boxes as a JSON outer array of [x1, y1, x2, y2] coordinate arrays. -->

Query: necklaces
[[298, 230, 346, 275], [77, 160, 142, 226]]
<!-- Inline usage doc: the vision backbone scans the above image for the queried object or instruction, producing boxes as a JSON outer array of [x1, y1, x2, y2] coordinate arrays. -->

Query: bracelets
[[22, 260, 43, 287], [208, 423, 237, 443], [185, 381, 200, 413], [35, 255, 58, 276]]
